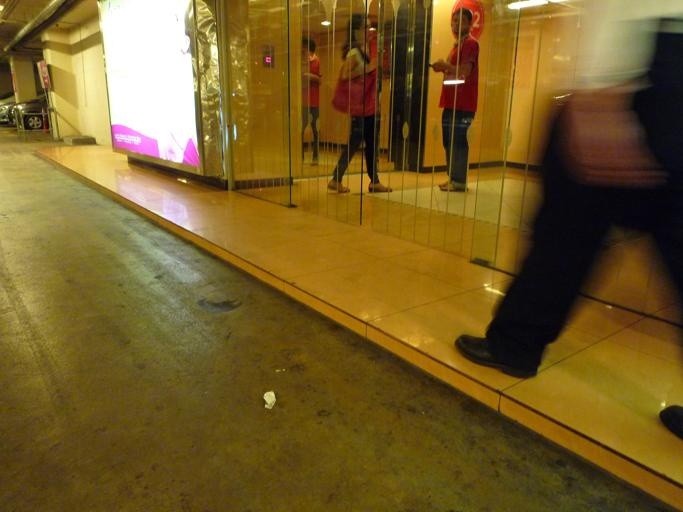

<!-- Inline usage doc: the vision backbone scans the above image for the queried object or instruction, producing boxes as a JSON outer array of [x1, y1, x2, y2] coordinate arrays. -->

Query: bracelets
[[358, 69, 363, 76]]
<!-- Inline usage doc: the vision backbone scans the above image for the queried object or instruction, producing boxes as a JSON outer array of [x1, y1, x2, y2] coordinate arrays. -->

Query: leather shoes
[[658, 405, 682, 437], [455, 334, 538, 380]]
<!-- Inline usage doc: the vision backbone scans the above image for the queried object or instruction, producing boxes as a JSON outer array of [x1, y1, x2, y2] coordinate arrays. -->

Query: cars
[[0, 88, 48, 126]]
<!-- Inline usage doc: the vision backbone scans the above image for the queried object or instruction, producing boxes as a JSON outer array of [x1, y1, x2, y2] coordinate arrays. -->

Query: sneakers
[[439, 181, 449, 188], [441, 183, 469, 192], [368, 183, 392, 192], [328, 179, 350, 193]]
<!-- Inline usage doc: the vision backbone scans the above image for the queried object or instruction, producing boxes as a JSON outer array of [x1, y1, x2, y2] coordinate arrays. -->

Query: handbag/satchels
[[332, 48, 379, 117]]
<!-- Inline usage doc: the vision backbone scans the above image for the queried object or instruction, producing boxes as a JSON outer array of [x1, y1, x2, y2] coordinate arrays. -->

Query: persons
[[430, 6, 479, 192], [299, 36, 321, 163], [455, 2, 680, 439], [326, 11, 392, 192]]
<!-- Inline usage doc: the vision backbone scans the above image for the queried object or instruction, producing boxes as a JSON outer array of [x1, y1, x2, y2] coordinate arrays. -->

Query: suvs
[[7, 94, 51, 130]]
